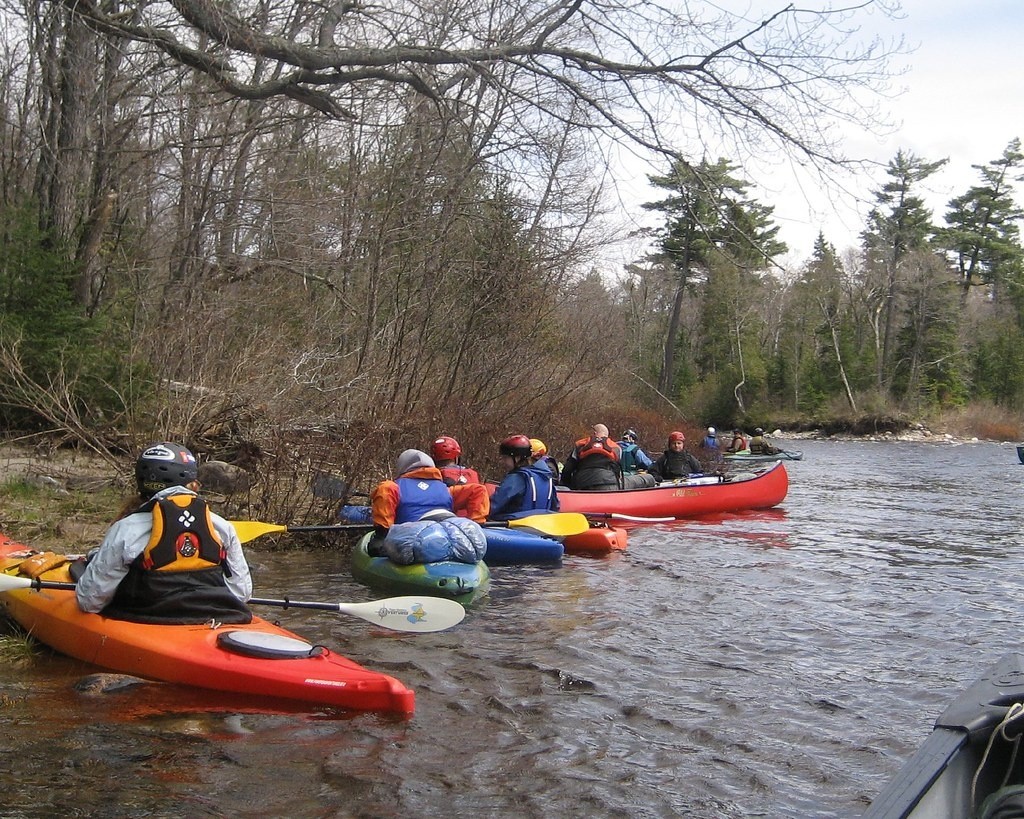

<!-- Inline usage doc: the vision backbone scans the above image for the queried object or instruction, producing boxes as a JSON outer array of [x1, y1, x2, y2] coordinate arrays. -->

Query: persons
[[76, 443, 252, 624], [749, 428, 781, 454], [488, 435, 561, 518], [559, 424, 655, 490], [726, 428, 746, 452], [368, 449, 489, 556], [615, 429, 652, 471], [699, 427, 722, 461], [647, 431, 700, 482], [431, 437, 479, 517]]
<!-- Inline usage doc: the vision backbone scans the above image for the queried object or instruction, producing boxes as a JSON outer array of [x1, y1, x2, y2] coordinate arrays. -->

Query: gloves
[[654, 476, 664, 483]]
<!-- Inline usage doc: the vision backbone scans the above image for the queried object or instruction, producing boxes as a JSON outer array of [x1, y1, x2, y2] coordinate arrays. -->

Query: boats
[[718, 448, 754, 456], [474, 459, 790, 516], [556, 521, 628, 553], [477, 524, 565, 563], [350, 528, 493, 598], [0, 531, 416, 713], [718, 449, 804, 464]]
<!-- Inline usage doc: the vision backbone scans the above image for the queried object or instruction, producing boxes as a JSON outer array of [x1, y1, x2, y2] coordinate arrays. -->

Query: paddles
[[0, 571, 468, 632], [582, 509, 676, 523], [782, 451, 800, 460], [226, 512, 591, 544]]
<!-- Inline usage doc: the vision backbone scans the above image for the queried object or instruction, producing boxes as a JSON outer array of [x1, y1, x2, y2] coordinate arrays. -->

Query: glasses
[[194, 478, 202, 491]]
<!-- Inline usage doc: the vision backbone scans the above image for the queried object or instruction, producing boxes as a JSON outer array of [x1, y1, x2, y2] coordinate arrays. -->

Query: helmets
[[733, 428, 742, 435], [429, 436, 461, 462], [528, 439, 547, 457], [753, 428, 764, 436], [707, 427, 716, 437], [669, 432, 685, 448], [499, 434, 532, 458], [622, 428, 638, 442], [134, 442, 198, 496]]
